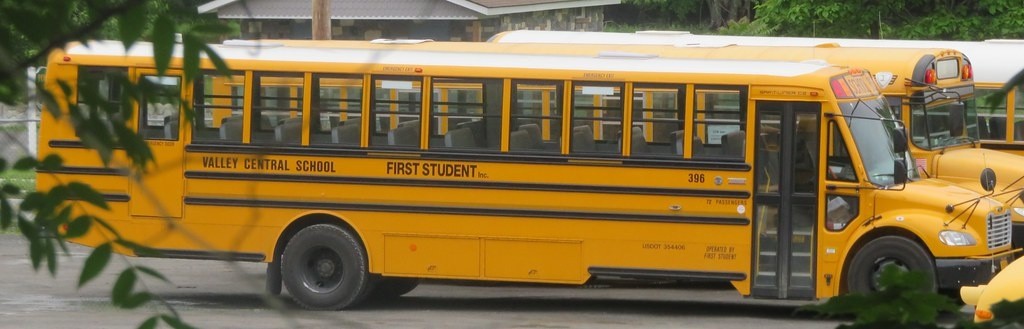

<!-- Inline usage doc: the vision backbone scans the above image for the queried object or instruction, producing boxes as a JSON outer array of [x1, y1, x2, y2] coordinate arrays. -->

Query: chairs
[[163, 111, 811, 191], [977, 116, 1024, 143]]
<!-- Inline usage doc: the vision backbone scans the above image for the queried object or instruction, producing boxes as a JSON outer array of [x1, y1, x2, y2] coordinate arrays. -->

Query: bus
[[37, 26, 1024, 315]]
